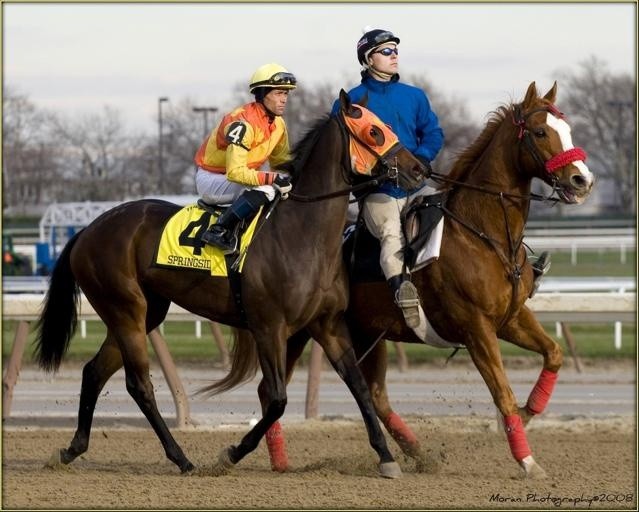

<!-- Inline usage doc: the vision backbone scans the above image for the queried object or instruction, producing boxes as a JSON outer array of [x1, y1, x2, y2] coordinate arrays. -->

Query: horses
[[192, 77, 598, 484], [31, 85, 430, 483]]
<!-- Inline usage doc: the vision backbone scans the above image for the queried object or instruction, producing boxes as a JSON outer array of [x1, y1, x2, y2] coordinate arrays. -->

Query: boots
[[529, 252, 551, 299], [389, 274, 420, 329], [204, 191, 256, 249]]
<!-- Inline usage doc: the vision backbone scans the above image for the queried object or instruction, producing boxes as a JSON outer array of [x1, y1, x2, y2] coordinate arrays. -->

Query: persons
[[331, 29, 445, 328], [194, 63, 297, 255]]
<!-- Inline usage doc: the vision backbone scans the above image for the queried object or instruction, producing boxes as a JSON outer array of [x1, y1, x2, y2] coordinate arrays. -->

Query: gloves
[[274, 173, 293, 192], [416, 155, 432, 179]]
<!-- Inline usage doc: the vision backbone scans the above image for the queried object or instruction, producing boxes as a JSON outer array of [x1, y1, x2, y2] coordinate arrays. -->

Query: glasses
[[374, 48, 399, 55], [271, 72, 295, 85]]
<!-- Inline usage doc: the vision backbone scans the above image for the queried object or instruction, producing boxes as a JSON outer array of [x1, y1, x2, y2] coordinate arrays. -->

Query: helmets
[[248, 64, 296, 93], [356, 29, 400, 55]]
